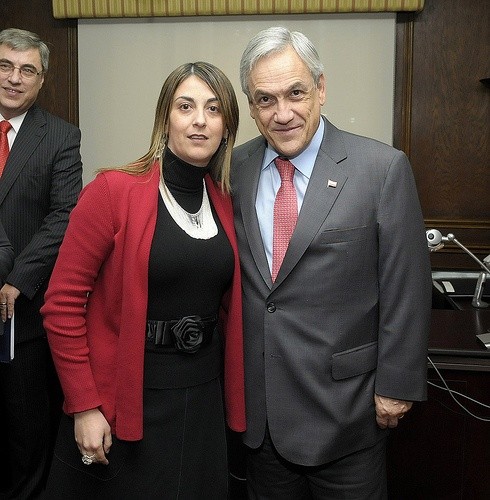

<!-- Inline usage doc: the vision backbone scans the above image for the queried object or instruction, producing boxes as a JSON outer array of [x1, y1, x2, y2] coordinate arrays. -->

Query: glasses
[[0, 62, 42, 79]]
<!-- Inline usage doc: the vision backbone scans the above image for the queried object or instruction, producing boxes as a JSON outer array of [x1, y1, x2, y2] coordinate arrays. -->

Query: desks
[[387, 308, 490, 500]]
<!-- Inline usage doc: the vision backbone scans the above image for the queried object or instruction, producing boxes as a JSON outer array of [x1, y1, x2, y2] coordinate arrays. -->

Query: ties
[[271, 157, 298, 286], [0, 120, 12, 179]]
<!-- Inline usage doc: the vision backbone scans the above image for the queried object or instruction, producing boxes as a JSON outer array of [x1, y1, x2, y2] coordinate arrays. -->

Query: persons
[[233, 26, 429, 500], [39, 60, 247, 500], [0, 28, 83, 500]]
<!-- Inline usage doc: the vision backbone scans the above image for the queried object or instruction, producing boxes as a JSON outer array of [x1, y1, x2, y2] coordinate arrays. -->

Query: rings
[[82, 453, 96, 466], [0, 303, 6, 305]]
